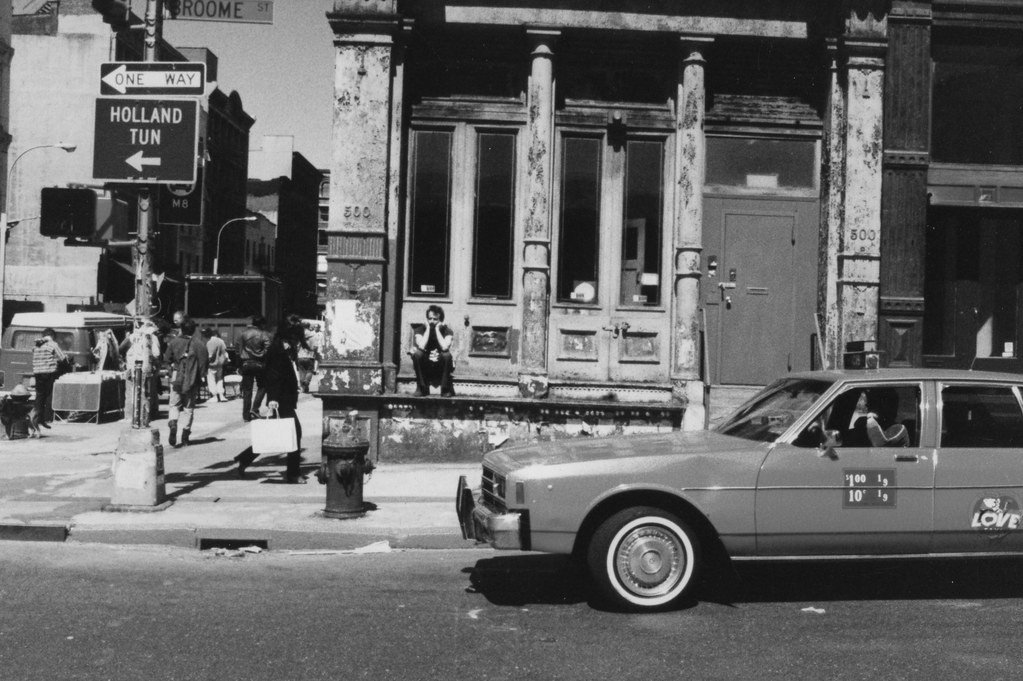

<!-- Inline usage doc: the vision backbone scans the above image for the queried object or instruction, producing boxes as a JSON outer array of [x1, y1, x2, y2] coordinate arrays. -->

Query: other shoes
[[244, 416, 252, 422], [304, 381, 309, 393], [441, 388, 452, 397], [220, 396, 228, 401], [250, 411, 261, 419], [206, 398, 218, 403], [414, 386, 430, 397]]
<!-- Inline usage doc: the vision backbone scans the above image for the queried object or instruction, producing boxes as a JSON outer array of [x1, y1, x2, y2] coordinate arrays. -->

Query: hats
[[287, 313, 301, 327]]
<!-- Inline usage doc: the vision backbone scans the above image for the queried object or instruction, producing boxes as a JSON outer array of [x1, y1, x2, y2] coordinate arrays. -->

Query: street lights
[[213, 216, 258, 276], [0, 143, 78, 298]]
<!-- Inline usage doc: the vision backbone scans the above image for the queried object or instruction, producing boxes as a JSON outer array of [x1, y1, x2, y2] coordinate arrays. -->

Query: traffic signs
[[92, 62, 207, 98], [92, 99, 198, 184]]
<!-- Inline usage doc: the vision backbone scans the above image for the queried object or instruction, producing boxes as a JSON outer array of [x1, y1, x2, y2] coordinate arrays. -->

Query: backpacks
[[172, 336, 202, 395]]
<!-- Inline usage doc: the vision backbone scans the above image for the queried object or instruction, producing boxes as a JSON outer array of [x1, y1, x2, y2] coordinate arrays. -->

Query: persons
[[235, 314, 311, 486], [238, 315, 271, 422], [163, 311, 209, 446], [205, 331, 230, 404], [840, 388, 913, 446], [407, 304, 459, 398], [31, 327, 68, 431]]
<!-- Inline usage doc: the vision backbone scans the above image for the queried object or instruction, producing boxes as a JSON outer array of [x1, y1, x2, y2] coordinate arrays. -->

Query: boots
[[168, 419, 177, 447], [182, 428, 191, 445]]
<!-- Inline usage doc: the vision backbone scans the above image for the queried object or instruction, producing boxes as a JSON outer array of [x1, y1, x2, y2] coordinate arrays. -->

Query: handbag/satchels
[[251, 405, 298, 454]]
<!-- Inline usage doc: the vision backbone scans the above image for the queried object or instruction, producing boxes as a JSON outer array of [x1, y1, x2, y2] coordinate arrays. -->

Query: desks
[[51, 377, 128, 427]]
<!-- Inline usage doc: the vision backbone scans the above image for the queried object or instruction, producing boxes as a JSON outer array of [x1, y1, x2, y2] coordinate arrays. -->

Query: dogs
[[0, 394, 52, 440]]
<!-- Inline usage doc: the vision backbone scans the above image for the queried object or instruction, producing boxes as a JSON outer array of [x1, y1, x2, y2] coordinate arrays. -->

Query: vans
[[0, 311, 160, 401]]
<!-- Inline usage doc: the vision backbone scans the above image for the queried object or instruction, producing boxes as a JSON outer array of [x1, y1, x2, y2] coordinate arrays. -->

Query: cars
[[455, 369, 1023, 614]]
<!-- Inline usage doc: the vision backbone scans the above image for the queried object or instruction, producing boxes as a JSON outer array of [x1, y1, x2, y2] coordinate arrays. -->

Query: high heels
[[287, 469, 307, 484], [234, 453, 252, 478]]
